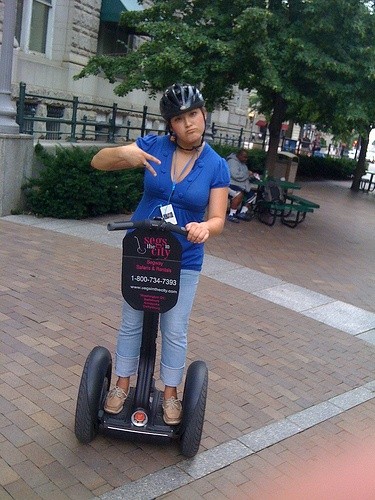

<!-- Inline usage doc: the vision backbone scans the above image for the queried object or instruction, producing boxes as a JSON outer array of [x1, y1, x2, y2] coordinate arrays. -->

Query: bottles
[[264, 168, 269, 181]]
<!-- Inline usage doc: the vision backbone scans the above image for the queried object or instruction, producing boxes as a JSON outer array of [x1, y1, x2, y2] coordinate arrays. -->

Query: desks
[[250, 174, 302, 214]]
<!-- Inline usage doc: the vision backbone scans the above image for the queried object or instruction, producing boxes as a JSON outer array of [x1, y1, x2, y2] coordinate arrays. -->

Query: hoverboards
[[74, 217, 209, 458]]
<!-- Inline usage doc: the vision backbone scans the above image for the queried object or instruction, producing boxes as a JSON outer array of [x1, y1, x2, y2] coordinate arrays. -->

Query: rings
[[143, 162, 147, 166]]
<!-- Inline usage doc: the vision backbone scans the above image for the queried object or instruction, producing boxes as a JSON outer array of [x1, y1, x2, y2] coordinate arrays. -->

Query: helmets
[[160, 83, 205, 124]]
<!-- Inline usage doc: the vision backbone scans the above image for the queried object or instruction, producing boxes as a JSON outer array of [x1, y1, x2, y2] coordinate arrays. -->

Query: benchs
[[226, 188, 320, 229], [358, 173, 375, 193]]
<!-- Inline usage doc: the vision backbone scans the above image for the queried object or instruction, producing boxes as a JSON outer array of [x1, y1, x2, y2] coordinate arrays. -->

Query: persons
[[91, 84, 229, 423], [225, 149, 260, 223]]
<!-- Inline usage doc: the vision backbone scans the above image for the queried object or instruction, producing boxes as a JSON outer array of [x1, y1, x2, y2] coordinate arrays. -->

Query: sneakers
[[162, 397, 182, 425], [226, 213, 239, 223], [236, 212, 251, 221], [104, 386, 130, 414]]
